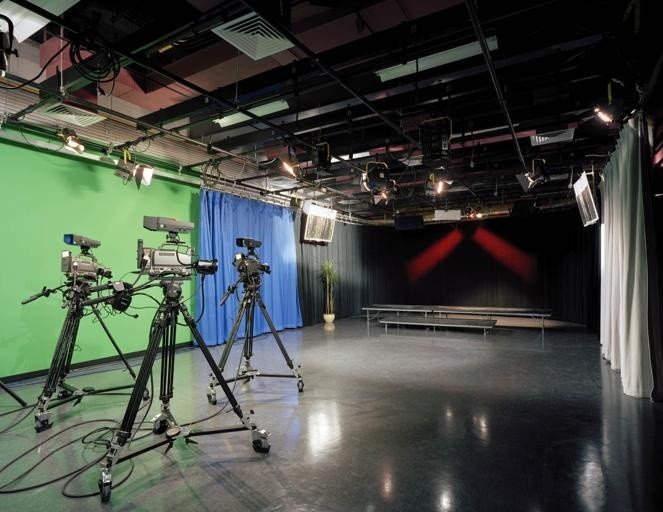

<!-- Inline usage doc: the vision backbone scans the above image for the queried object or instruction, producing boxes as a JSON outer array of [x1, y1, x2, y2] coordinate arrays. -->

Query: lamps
[[114, 158, 152, 191], [62, 128, 85, 153], [423, 167, 446, 197], [594, 77, 642, 127], [360, 161, 390, 193]]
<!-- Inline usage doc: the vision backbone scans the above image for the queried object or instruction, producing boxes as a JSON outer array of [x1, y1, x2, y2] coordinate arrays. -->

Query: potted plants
[[317, 259, 338, 323]]
